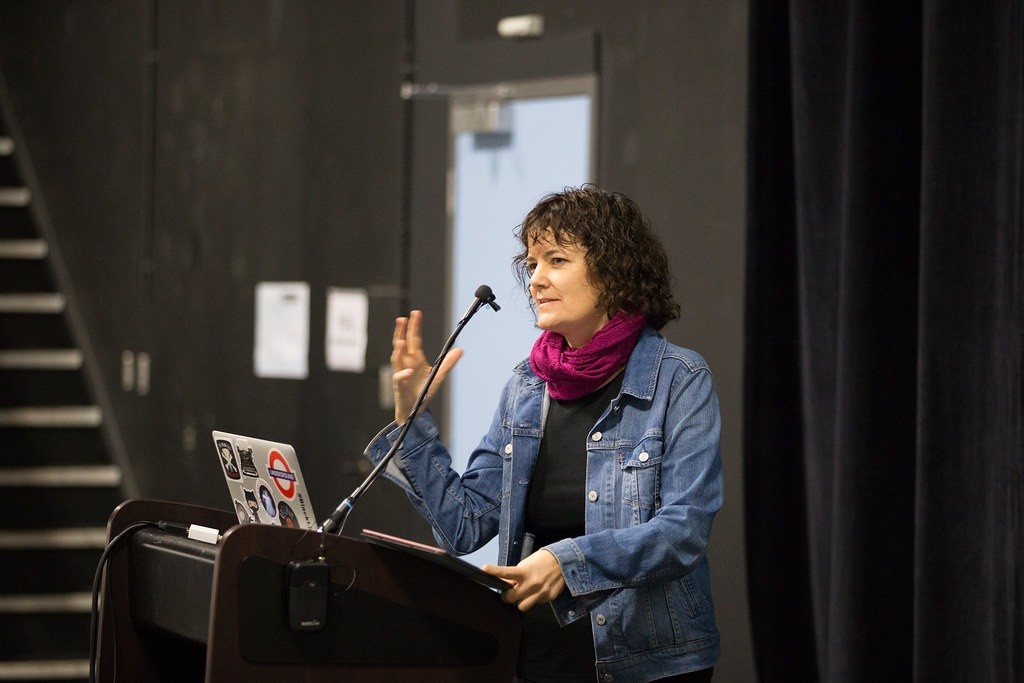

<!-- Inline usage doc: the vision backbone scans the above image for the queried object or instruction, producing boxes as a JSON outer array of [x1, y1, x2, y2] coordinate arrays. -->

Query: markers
[[520, 532, 536, 561]]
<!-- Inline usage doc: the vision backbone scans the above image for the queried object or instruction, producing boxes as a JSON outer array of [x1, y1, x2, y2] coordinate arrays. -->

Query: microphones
[[316, 284, 492, 533]]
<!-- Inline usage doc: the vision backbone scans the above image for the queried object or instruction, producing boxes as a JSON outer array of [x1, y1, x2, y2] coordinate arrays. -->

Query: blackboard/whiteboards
[[444, 73, 601, 574]]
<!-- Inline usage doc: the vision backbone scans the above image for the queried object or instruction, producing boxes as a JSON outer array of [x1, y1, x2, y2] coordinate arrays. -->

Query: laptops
[[211, 430, 318, 532]]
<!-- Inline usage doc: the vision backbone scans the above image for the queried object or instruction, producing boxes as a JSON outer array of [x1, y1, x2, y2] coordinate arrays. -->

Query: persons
[[363, 182, 725, 683]]
